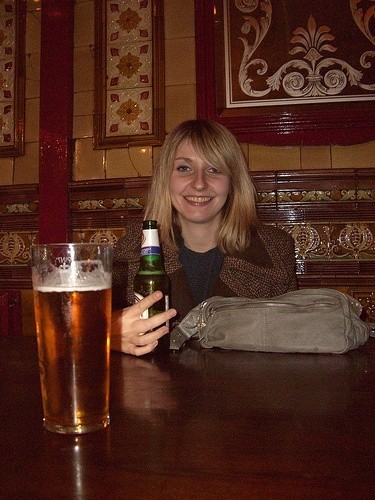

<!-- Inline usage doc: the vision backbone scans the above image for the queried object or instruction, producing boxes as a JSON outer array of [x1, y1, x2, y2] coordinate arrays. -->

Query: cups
[[29, 242, 112, 433]]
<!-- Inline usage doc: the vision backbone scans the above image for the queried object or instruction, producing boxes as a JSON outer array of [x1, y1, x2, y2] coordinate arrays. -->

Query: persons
[[88, 119, 298, 355]]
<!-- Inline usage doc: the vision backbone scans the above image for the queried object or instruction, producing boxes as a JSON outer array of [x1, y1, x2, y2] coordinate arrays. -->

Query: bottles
[[132, 219, 172, 347]]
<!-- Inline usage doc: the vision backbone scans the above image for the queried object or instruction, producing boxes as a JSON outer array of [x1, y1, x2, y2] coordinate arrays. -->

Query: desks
[[0, 335, 375, 500]]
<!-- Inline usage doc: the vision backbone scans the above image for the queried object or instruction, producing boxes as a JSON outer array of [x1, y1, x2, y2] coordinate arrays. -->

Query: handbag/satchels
[[171, 288, 368, 353]]
